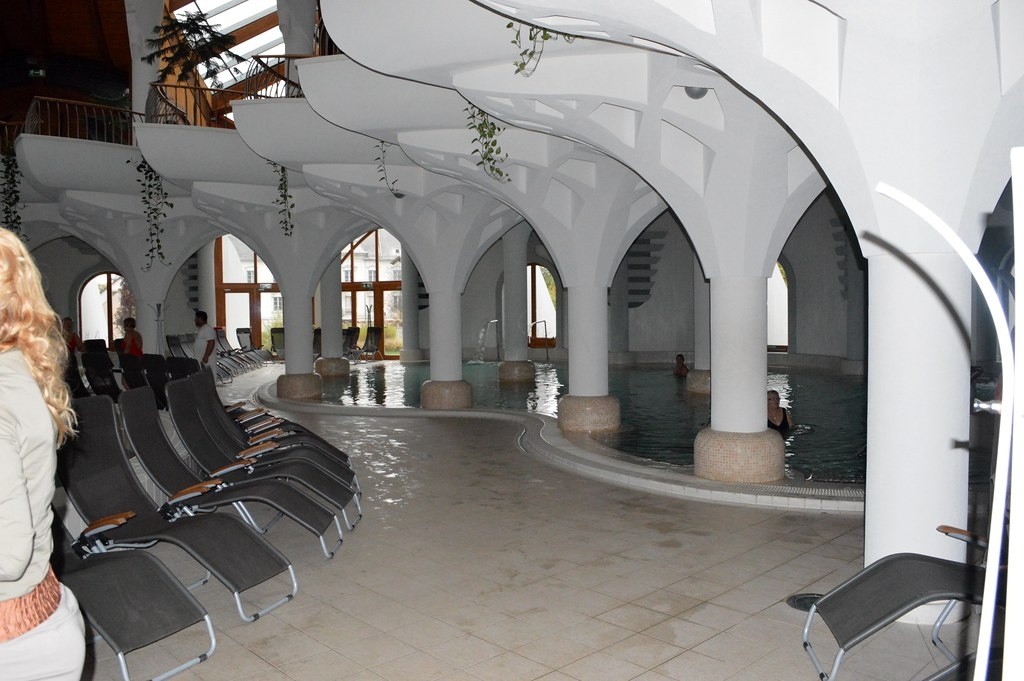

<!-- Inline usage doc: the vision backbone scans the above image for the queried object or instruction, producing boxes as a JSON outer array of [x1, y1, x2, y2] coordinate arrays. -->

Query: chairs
[[50, 326, 384, 681], [802, 523, 1010, 681]]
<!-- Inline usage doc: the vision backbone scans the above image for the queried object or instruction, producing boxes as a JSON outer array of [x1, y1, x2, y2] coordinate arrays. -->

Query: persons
[[192, 311, 217, 385], [120, 318, 143, 359], [768, 390, 794, 438], [1, 229, 86, 680], [61, 317, 90, 399], [674, 354, 689, 377]]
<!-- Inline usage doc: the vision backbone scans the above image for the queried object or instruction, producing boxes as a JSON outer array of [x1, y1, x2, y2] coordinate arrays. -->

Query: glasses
[[768, 397, 781, 401]]
